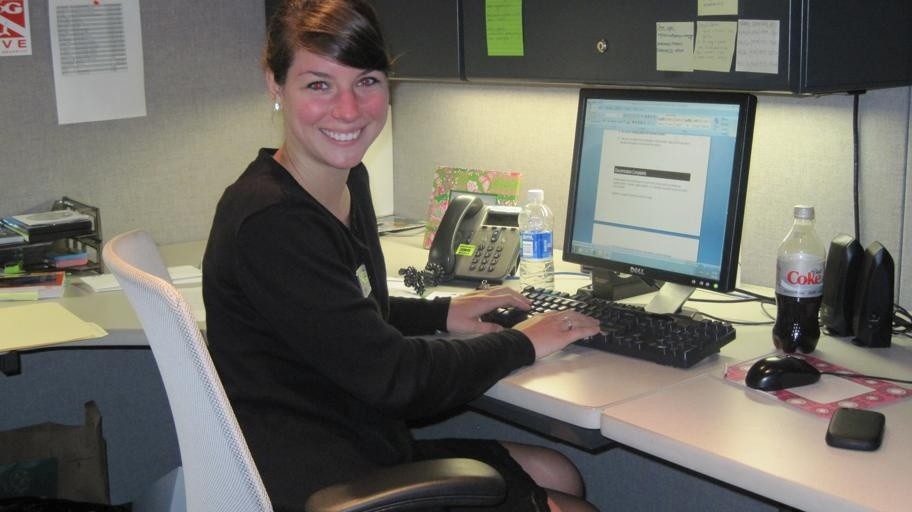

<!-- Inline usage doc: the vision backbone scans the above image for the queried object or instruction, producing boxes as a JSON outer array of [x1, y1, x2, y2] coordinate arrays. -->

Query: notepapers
[[45, 247, 89, 268]]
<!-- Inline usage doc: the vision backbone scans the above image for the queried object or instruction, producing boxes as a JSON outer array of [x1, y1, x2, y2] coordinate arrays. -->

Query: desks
[[601, 322, 912, 512], [1, 214, 822, 512]]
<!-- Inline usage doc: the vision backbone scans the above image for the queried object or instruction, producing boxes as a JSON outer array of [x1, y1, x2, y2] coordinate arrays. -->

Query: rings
[[562, 316, 574, 330]]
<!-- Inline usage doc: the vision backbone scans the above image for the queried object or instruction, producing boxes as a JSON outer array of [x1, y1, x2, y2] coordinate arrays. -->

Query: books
[[1, 209, 203, 303], [420, 163, 522, 253]]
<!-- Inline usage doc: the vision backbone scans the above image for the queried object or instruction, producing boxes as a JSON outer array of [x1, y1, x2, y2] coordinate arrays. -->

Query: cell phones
[[826, 407, 885, 450]]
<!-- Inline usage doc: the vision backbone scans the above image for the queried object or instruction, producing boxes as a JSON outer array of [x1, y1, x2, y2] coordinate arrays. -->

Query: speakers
[[851, 241, 894, 347], [820, 233, 865, 336]]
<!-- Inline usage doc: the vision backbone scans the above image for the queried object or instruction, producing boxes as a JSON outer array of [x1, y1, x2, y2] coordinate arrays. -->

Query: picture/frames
[[423, 167, 518, 248]]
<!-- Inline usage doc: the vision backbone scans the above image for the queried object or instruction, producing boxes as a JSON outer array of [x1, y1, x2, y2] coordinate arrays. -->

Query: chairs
[[102, 231, 507, 512]]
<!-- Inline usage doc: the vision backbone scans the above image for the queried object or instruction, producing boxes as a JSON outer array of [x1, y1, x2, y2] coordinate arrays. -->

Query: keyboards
[[480, 285, 736, 369]]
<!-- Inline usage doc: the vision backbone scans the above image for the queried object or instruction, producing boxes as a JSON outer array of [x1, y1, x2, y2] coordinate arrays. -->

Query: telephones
[[429, 195, 522, 280]]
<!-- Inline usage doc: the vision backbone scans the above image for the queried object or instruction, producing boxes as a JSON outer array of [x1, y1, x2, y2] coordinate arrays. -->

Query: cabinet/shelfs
[[372, 0, 912, 99]]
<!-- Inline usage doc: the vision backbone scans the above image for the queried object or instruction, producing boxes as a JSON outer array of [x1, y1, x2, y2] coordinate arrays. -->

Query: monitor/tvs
[[562, 88, 757, 319]]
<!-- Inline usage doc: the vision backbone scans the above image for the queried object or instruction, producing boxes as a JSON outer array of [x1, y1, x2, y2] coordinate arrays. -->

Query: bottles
[[772, 205, 828, 354], [517, 187, 555, 295]]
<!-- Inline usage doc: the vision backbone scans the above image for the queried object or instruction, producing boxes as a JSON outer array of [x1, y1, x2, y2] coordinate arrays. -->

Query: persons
[[198, 0, 598, 512]]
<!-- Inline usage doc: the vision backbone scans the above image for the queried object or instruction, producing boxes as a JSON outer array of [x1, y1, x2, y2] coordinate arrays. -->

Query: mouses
[[745, 355, 821, 390]]
